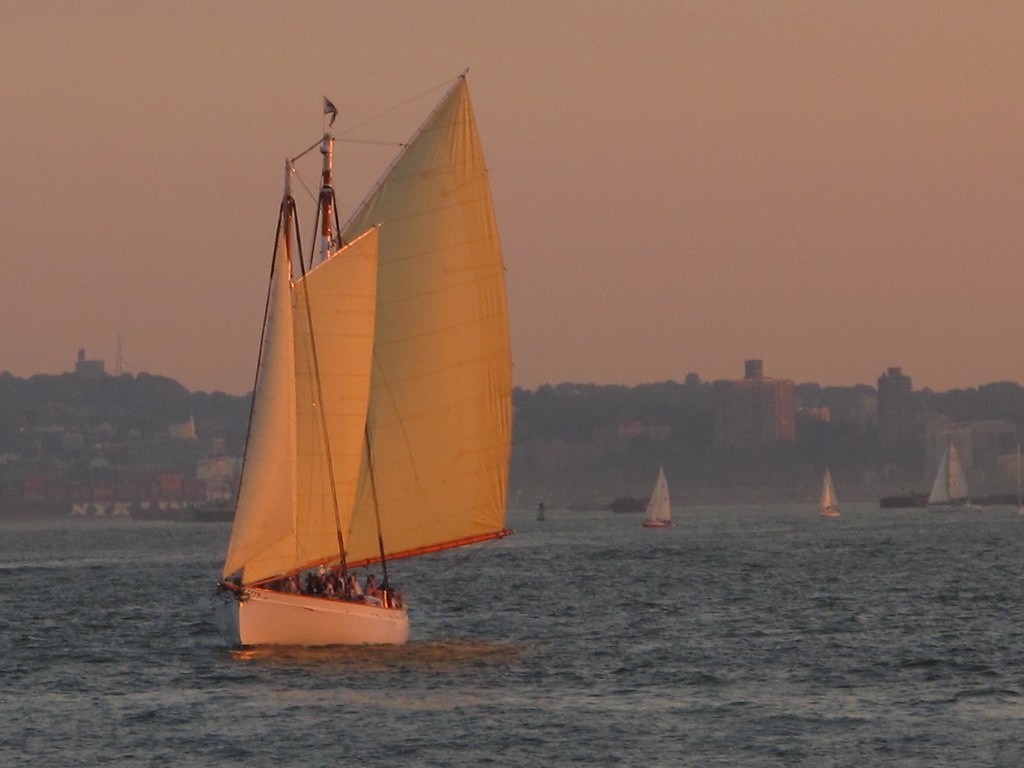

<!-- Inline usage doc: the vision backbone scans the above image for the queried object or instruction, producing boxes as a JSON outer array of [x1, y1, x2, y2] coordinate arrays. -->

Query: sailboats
[[219, 64, 516, 647], [816, 468, 845, 519], [639, 463, 673, 527], [927, 441, 981, 512]]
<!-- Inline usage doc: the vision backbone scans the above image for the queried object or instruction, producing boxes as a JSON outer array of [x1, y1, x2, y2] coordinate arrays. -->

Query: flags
[[323, 97, 338, 128]]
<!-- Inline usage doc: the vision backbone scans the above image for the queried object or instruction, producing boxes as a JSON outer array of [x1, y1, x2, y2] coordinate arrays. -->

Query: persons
[[277, 572, 302, 594], [305, 573, 365, 601], [365, 575, 396, 607]]
